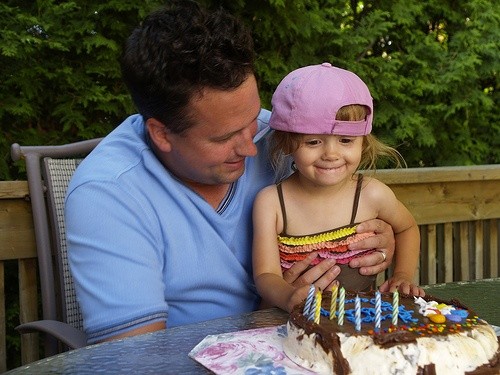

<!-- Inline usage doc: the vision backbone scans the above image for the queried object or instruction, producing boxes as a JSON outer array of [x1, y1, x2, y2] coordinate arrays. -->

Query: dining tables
[[0, 275, 500, 375]]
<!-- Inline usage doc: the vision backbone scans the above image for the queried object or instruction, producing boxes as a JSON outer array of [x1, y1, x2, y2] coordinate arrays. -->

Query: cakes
[[284, 290, 500, 375]]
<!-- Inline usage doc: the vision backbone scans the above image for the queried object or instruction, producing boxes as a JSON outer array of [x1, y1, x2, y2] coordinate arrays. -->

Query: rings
[[376, 250, 386, 262]]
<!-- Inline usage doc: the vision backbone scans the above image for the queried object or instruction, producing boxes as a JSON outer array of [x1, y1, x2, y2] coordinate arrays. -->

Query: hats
[[253, 62, 373, 144]]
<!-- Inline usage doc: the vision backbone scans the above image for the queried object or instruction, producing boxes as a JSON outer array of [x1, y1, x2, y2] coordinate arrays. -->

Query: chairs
[[10, 137, 103, 350]]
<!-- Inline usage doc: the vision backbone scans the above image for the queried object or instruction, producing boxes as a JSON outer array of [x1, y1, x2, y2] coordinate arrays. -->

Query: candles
[[375, 286, 382, 328], [303, 283, 324, 325], [355, 293, 362, 331], [392, 286, 399, 326], [329, 282, 338, 321], [338, 284, 346, 325]]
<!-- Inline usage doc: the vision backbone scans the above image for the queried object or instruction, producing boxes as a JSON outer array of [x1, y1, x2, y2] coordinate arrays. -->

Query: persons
[[63, 5, 396, 344], [253, 63, 425, 313]]
